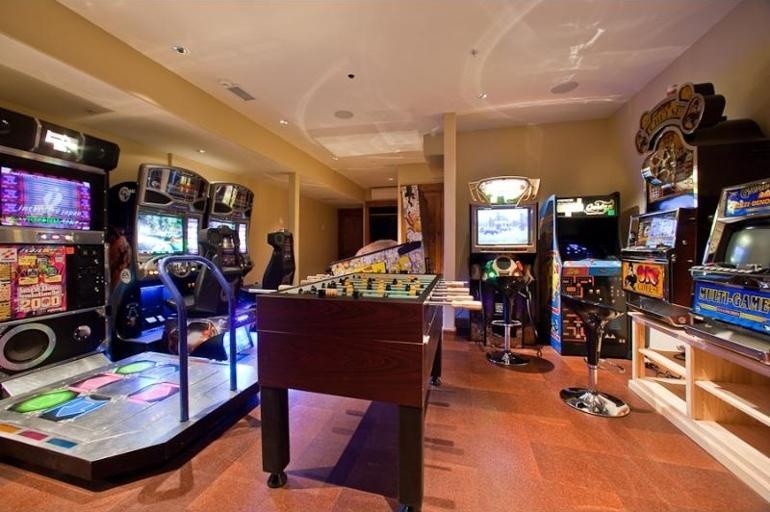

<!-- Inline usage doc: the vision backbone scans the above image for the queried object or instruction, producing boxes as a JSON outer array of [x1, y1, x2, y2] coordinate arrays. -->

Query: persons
[[106, 229, 131, 287]]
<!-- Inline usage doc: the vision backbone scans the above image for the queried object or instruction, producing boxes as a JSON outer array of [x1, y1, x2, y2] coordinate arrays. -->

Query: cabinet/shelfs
[[627, 310, 770, 502]]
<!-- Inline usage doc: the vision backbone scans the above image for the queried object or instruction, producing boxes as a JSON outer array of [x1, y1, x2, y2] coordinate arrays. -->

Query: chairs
[[164, 224, 243, 317], [241, 230, 295, 302]]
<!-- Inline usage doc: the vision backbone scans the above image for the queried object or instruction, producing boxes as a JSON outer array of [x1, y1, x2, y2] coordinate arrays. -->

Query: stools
[[559, 292, 631, 418], [486, 275, 530, 365]]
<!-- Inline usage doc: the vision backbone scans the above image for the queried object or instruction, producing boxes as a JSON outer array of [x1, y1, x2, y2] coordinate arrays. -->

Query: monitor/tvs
[[477, 207, 531, 246], [722, 221, 770, 272], [207, 219, 247, 255], [0, 163, 95, 229], [137, 212, 185, 254]]
